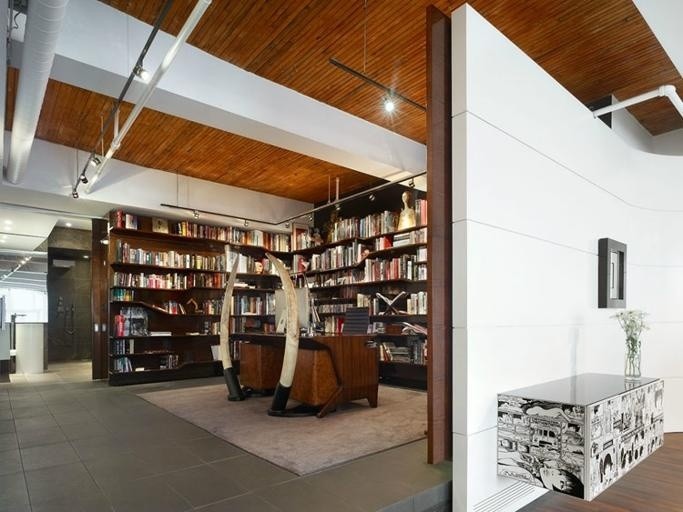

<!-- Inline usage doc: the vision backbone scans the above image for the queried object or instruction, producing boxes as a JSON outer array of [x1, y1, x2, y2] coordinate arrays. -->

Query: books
[[290, 198, 429, 366], [109, 208, 291, 373]]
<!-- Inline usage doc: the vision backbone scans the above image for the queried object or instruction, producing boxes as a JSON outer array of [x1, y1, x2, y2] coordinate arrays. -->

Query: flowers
[[607, 307, 651, 374]]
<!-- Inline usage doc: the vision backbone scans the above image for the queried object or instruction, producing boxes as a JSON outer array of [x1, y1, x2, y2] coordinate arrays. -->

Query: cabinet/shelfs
[[107, 209, 292, 386], [292, 226, 427, 392]]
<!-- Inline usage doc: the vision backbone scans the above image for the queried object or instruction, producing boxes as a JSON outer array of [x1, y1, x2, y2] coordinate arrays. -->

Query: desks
[[230, 328, 378, 418]]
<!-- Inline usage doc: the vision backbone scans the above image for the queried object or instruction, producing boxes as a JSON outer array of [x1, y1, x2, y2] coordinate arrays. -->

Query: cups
[[623, 347, 641, 382]]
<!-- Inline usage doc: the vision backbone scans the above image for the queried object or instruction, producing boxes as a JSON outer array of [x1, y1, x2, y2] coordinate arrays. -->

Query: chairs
[[341, 307, 369, 333]]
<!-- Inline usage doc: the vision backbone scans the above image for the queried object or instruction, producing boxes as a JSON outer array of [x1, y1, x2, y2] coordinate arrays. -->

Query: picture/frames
[[598, 236, 626, 308]]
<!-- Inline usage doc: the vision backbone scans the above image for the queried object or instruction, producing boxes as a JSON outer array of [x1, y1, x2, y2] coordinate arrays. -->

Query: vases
[[624, 340, 642, 380]]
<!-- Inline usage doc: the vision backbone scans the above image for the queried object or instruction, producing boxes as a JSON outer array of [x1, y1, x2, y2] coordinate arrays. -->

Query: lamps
[[160, 204, 275, 227], [328, 56, 426, 115], [275, 169, 426, 226], [70, 0, 172, 199]]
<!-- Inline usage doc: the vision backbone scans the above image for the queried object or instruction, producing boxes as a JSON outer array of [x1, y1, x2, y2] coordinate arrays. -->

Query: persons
[[398, 190, 417, 231]]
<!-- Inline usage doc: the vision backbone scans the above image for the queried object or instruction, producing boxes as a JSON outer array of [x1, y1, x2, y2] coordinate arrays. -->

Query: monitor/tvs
[[275, 288, 309, 335]]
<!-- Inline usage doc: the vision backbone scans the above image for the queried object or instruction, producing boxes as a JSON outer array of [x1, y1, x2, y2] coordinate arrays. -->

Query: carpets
[[137, 383, 427, 478]]
